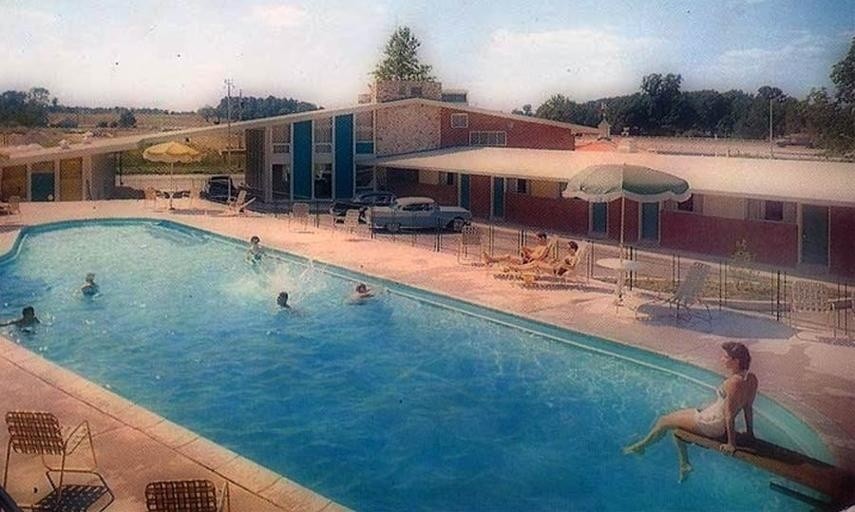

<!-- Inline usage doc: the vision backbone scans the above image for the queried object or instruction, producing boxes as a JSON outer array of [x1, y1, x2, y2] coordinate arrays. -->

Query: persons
[[83, 271, 99, 294], [482, 227, 549, 267], [246, 235, 265, 265], [497, 237, 580, 277], [0, 304, 41, 337], [345, 282, 377, 304], [620, 341, 761, 483], [273, 289, 289, 307]]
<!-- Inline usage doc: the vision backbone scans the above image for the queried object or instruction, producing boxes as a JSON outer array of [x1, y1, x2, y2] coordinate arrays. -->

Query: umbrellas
[[141, 142, 202, 207], [561, 162, 691, 298]]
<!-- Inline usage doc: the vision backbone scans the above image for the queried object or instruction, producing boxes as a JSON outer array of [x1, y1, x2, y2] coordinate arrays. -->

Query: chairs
[[470, 234, 591, 290], [633, 262, 713, 323], [4, 409, 97, 508], [788, 279, 838, 340], [285, 203, 360, 242], [0, 195, 23, 226], [456, 226, 482, 265], [141, 179, 257, 217], [145, 479, 230, 512]]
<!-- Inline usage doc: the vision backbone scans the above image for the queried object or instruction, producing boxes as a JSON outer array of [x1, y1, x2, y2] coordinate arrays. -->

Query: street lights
[[769, 95, 776, 152]]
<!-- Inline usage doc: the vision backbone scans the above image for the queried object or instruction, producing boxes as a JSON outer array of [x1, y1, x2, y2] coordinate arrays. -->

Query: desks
[[596, 258, 638, 306]]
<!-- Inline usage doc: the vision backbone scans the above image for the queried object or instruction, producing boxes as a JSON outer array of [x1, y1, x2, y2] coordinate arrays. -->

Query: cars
[[204, 176, 236, 202], [331, 191, 473, 233]]
